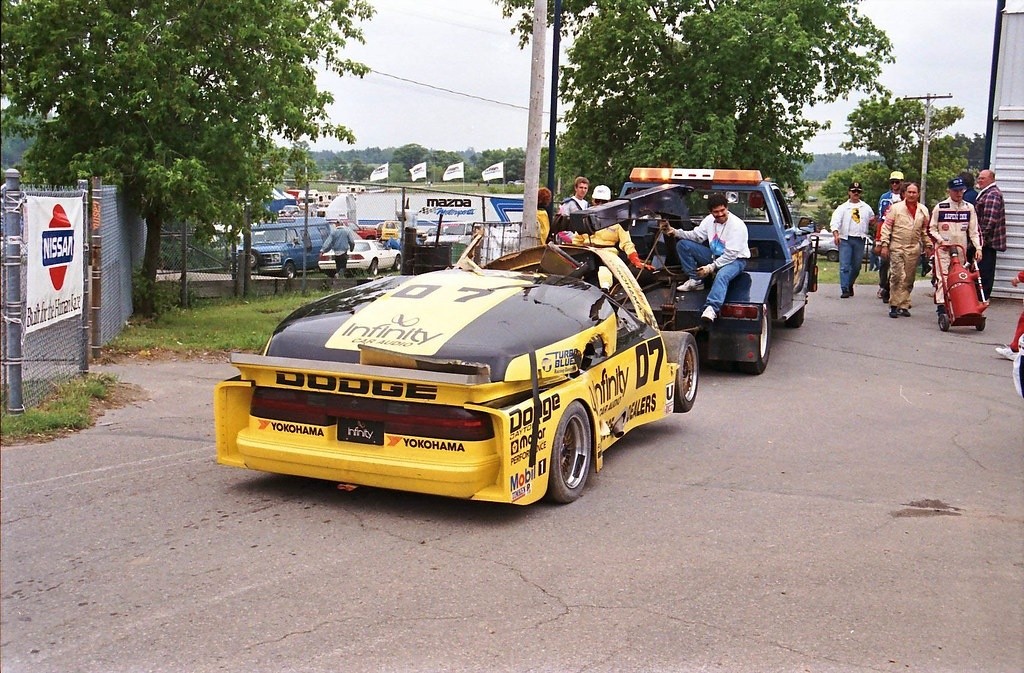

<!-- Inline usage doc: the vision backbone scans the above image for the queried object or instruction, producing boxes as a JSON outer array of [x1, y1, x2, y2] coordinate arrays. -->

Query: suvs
[[231, 217, 331, 279]]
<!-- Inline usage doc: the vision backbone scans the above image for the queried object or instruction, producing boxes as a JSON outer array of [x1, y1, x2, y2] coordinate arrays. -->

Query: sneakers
[[677, 279, 705, 292], [995, 344, 1018, 361], [700, 305, 717, 323]]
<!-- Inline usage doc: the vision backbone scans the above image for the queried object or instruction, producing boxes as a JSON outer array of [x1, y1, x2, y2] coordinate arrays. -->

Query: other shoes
[[883, 292, 890, 303], [987, 299, 989, 305], [889, 306, 899, 318], [876, 288, 883, 298], [850, 288, 854, 296], [936, 305, 946, 316], [899, 309, 910, 317], [841, 291, 849, 298]]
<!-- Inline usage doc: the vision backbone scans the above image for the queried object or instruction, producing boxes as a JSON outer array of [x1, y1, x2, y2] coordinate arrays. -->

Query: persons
[[557, 176, 588, 215], [660, 193, 751, 323], [994, 271, 1024, 361], [574, 185, 656, 270], [319, 220, 355, 278], [830, 183, 876, 299], [873, 170, 1007, 322], [538, 187, 552, 246]]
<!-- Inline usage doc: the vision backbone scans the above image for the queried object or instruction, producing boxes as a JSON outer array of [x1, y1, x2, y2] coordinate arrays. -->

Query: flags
[[369, 163, 388, 182], [443, 162, 464, 181], [409, 161, 427, 182], [482, 162, 504, 181]]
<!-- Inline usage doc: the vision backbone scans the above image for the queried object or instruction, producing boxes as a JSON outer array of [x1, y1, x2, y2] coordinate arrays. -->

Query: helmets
[[889, 171, 903, 180], [592, 185, 612, 200]]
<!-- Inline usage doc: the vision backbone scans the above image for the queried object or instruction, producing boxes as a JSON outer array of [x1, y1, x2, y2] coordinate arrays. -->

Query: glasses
[[595, 199, 608, 204], [891, 180, 900, 184], [851, 190, 862, 194]]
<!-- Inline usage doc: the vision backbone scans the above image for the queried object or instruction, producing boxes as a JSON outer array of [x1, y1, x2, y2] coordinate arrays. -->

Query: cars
[[807, 196, 817, 203], [810, 232, 875, 262], [317, 238, 402, 275], [332, 218, 476, 251]]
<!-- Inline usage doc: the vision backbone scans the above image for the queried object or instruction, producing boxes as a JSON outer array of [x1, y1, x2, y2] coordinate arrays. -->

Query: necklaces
[[713, 221, 726, 253]]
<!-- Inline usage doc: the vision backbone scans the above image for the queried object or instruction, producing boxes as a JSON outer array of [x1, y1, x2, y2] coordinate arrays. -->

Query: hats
[[849, 183, 862, 192], [948, 177, 966, 190]]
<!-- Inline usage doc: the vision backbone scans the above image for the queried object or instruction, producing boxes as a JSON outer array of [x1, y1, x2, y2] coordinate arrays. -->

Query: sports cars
[[213, 238, 701, 510]]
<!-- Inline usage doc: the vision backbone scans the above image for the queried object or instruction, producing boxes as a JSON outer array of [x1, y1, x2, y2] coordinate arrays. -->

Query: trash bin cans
[[414, 245, 452, 275]]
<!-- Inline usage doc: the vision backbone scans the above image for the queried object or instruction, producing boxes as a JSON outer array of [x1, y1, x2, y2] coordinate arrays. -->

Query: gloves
[[660, 220, 675, 238], [697, 264, 715, 279], [628, 252, 656, 272]]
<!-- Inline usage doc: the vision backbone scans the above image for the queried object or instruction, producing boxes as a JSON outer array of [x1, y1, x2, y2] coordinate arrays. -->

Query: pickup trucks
[[597, 165, 824, 375]]
